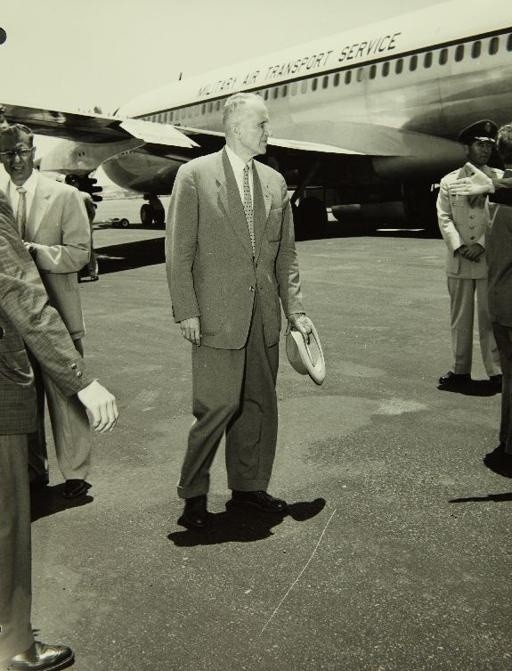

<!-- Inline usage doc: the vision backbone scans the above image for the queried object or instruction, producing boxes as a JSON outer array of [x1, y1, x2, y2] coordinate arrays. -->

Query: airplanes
[[0, 1, 512, 238]]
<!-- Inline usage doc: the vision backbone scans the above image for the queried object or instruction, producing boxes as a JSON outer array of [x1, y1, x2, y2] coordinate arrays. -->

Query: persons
[[481, 121, 511, 471], [0, 28, 119, 671], [164, 93, 312, 533], [65, 172, 100, 281], [449, 161, 512, 208], [0, 121, 91, 500], [436, 120, 501, 393]]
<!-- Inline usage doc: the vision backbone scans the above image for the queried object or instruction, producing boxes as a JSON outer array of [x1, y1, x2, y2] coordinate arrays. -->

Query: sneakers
[[479, 374, 503, 395]]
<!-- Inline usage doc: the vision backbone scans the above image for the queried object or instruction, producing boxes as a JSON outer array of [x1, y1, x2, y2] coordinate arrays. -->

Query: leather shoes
[[230, 490, 287, 515], [181, 495, 207, 527], [439, 371, 471, 386], [8, 640, 75, 671], [63, 480, 86, 496]]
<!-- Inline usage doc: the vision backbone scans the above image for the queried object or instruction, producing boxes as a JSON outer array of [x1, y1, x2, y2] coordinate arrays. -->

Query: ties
[[15, 188, 27, 238], [243, 163, 256, 256]]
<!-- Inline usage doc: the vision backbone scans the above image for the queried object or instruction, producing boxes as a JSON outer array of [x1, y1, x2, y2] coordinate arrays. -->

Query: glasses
[[0, 146, 35, 157]]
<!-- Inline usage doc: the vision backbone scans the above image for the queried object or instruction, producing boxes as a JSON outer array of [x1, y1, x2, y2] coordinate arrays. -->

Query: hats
[[460, 118, 499, 144], [285, 316, 328, 386]]
